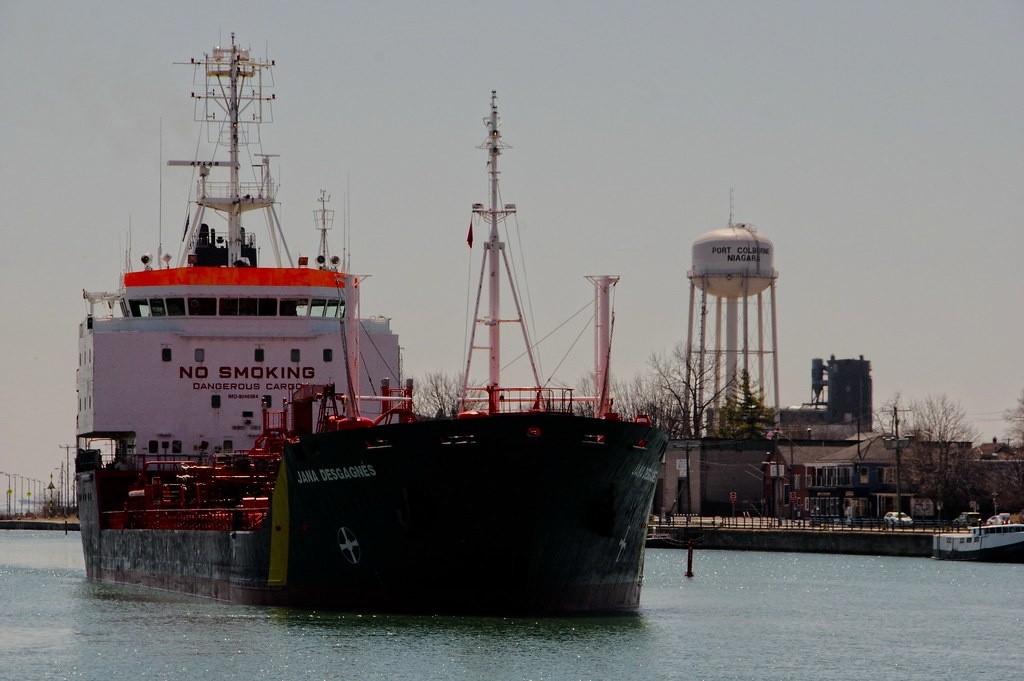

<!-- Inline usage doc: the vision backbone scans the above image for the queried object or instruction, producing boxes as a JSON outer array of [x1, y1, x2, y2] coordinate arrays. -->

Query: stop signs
[[790, 491, 797, 499], [730, 492, 737, 499]]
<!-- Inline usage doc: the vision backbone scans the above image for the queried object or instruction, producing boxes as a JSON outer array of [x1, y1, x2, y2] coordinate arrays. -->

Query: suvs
[[954, 512, 981, 528]]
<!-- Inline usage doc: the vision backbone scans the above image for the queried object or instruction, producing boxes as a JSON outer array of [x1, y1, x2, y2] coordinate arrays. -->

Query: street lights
[[14, 473, 23, 516], [55, 467, 67, 515], [26, 477, 46, 514], [882, 407, 910, 513], [5, 474, 17, 515], [0, 471, 11, 519]]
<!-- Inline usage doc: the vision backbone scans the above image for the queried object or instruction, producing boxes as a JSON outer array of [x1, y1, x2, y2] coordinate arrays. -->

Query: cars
[[883, 512, 914, 528], [985, 514, 1011, 525]]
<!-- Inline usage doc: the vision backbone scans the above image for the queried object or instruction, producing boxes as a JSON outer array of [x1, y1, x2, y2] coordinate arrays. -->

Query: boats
[[71, 33, 675, 619]]
